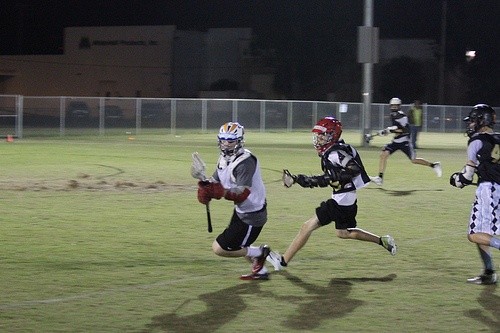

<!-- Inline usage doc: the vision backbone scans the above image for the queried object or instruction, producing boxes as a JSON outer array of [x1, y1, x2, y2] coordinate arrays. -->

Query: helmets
[[312, 116, 342, 155], [217, 122, 244, 162], [462, 104, 496, 138], [390, 98, 401, 112]]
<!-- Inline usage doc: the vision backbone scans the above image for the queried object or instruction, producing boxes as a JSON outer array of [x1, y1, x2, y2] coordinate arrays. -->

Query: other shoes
[[369, 176, 383, 186], [433, 162, 442, 177], [467, 273, 497, 285]]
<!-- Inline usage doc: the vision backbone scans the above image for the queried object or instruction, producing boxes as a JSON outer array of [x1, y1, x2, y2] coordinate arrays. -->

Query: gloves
[[296, 174, 318, 189], [198, 180, 224, 200], [197, 187, 212, 204], [382, 127, 393, 135], [325, 168, 343, 189], [450, 172, 472, 189]]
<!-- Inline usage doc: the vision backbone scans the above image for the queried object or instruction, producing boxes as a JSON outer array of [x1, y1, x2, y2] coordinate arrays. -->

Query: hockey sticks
[[468, 183, 477, 186], [282, 168, 298, 190], [364, 129, 402, 144], [190, 151, 213, 233]]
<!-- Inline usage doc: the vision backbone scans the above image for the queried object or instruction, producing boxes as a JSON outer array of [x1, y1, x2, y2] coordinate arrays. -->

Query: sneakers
[[381, 235, 397, 255], [240, 272, 270, 281], [265, 250, 287, 271], [251, 245, 271, 273]]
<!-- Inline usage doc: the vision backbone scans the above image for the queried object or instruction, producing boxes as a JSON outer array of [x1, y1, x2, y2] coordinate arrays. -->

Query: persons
[[197, 123, 271, 280], [266, 116, 397, 269], [408, 99, 422, 149], [370, 98, 441, 185], [450, 104, 500, 283]]
